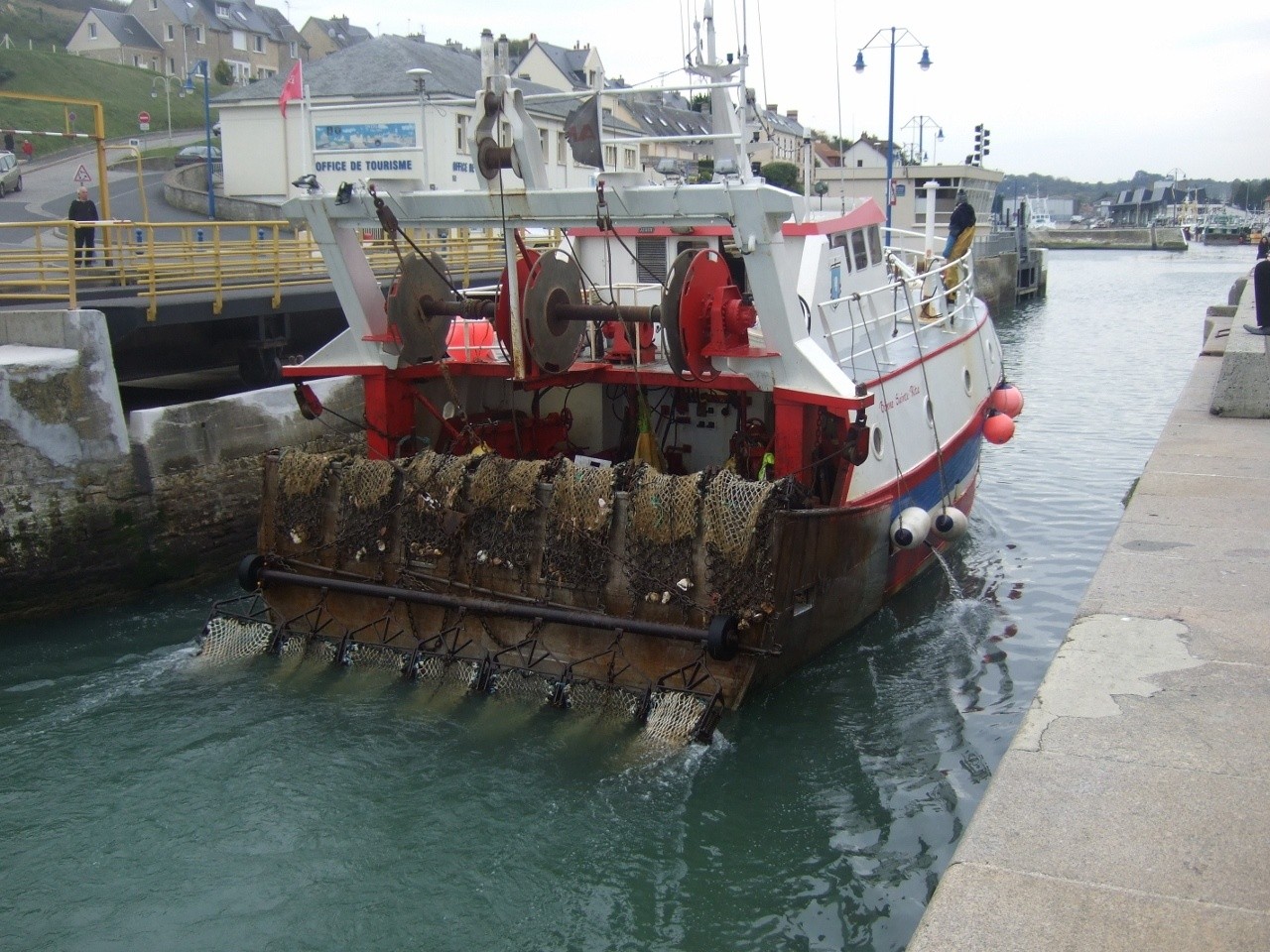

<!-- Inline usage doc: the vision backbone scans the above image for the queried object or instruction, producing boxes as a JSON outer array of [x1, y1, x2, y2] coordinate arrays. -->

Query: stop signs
[[138, 112, 149, 123]]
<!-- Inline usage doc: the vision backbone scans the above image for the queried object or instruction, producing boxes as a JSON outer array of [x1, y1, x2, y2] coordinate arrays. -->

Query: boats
[[180, 0, 1024, 776], [1027, 178, 1056, 229]]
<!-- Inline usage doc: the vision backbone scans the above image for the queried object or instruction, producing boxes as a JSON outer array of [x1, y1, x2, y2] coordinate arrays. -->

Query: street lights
[[151, 76, 185, 140], [854, 25, 933, 249], [900, 115, 944, 167], [184, 58, 215, 222], [1165, 167, 1185, 229]]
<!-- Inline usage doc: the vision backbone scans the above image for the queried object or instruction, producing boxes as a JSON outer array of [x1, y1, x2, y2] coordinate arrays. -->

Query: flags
[[562, 94, 604, 171], [279, 60, 301, 117]]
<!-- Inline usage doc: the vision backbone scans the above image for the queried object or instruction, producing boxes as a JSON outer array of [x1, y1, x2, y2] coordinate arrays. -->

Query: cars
[[213, 121, 221, 136], [174, 146, 222, 168], [447, 227, 567, 253], [0, 150, 23, 198]]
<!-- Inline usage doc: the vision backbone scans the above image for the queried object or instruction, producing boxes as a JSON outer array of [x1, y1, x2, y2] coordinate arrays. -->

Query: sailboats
[[1171, 186, 1270, 245]]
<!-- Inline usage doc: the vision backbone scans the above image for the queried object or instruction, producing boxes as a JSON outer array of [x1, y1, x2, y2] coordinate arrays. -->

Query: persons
[[68, 186, 98, 268], [942, 188, 976, 302], [22, 139, 33, 165], [1243, 261, 1270, 336], [1257, 236, 1270, 263], [5, 133, 15, 153]]
